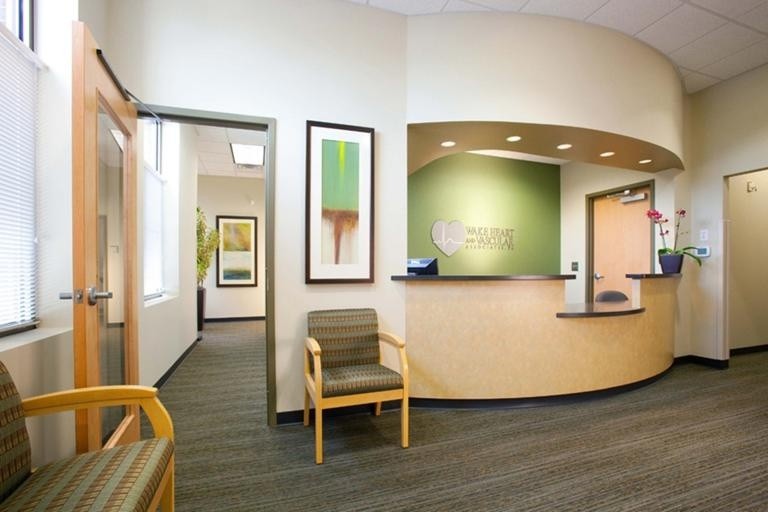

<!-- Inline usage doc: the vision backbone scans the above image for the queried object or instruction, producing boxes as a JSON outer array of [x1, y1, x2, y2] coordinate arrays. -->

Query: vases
[[657, 253, 684, 274]]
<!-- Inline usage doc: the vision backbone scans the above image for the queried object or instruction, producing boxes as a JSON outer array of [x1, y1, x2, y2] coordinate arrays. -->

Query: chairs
[[1, 361, 178, 512], [303, 308, 409, 464], [597, 290, 629, 301]]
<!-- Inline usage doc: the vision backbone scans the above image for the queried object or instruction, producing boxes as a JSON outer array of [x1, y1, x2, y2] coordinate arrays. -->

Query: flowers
[[645, 208, 710, 267]]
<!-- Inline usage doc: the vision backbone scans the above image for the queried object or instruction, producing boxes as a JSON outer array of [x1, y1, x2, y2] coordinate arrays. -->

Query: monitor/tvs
[[407, 257, 438, 276]]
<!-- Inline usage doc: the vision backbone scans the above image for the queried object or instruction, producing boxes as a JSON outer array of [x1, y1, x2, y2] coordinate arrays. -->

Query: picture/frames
[[215, 215, 258, 287], [305, 119, 374, 282]]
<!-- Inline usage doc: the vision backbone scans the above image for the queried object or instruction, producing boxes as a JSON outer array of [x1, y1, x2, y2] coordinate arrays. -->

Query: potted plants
[[197, 207, 220, 331]]
